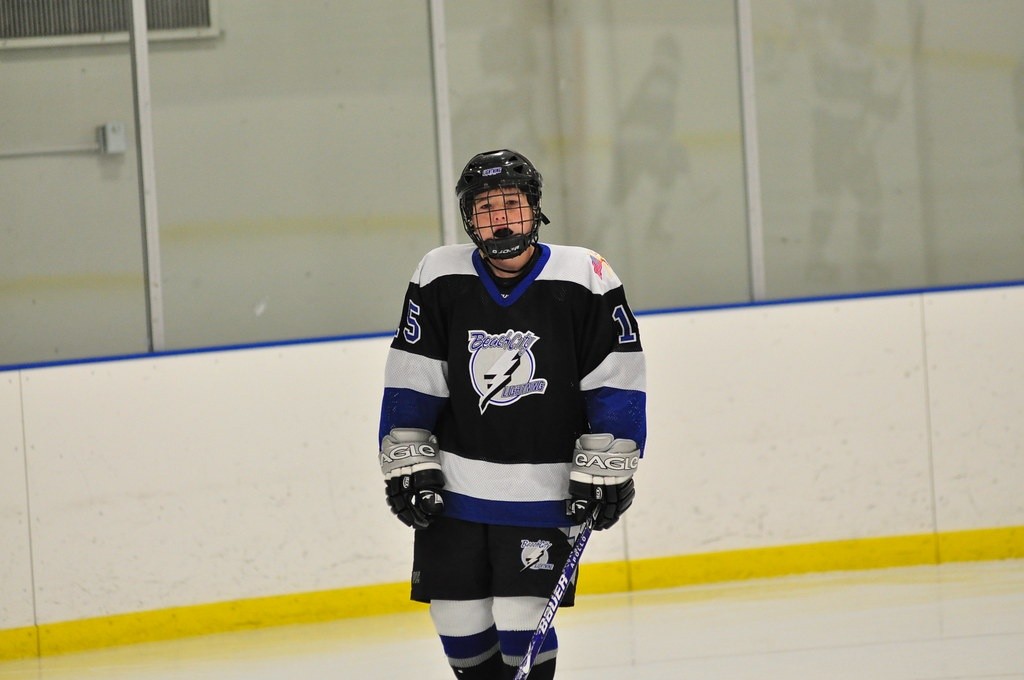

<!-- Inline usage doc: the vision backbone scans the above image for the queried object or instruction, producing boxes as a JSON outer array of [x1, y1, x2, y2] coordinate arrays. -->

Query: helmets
[[455, 148, 542, 259]]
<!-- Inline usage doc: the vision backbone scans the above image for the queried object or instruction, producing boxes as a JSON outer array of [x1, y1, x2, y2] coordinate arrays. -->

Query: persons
[[377, 150, 647, 680]]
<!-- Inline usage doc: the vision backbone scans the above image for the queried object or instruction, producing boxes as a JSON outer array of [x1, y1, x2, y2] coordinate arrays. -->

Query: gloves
[[569, 433, 641, 531], [378, 428, 446, 530]]
